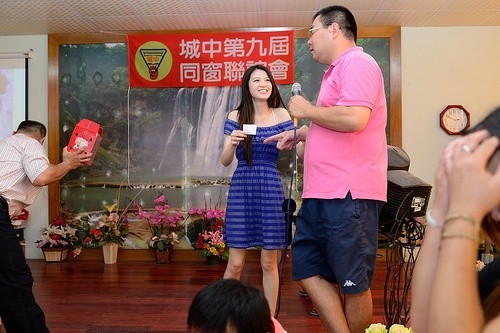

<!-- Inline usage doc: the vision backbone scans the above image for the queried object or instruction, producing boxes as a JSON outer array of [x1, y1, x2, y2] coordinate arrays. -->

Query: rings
[[461, 145, 472, 153]]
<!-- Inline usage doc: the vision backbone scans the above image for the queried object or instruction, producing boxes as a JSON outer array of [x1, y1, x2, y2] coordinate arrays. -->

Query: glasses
[[308, 26, 326, 38]]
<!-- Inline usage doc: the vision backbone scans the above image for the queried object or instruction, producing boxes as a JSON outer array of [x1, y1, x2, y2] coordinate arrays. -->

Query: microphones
[[290, 83, 301, 128]]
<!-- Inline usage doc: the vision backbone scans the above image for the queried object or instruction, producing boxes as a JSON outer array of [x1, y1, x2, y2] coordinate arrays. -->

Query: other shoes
[[309, 308, 319, 315], [298, 290, 309, 297]]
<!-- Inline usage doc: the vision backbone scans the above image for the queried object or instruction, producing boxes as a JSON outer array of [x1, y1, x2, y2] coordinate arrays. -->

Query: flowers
[[34, 216, 85, 258], [187, 196, 230, 260], [82, 200, 129, 247], [133, 189, 187, 252], [365, 322, 414, 333]]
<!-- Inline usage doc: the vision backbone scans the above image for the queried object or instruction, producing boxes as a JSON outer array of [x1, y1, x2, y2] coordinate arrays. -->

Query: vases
[[102, 243, 119, 264], [206, 254, 222, 265], [40, 247, 68, 263], [152, 248, 173, 265]]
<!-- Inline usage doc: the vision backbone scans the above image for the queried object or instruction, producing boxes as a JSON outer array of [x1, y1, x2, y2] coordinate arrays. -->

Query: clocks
[[439, 104, 471, 135]]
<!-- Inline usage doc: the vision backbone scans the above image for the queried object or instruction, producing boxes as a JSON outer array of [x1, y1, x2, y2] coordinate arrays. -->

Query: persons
[[410, 104, 500, 333], [263, 5, 388, 333], [0, 120, 93, 333], [299, 289, 319, 316], [220, 65, 295, 317], [185, 278, 275, 333]]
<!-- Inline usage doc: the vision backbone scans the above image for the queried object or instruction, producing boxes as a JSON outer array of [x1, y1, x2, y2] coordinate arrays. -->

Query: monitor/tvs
[[381, 145, 433, 219]]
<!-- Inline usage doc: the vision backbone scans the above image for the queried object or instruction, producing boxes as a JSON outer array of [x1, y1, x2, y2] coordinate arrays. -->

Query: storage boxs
[[67, 118, 103, 166]]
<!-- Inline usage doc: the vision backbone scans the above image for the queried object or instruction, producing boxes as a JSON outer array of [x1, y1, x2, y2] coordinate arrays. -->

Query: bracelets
[[424, 210, 444, 229], [440, 214, 482, 243]]
